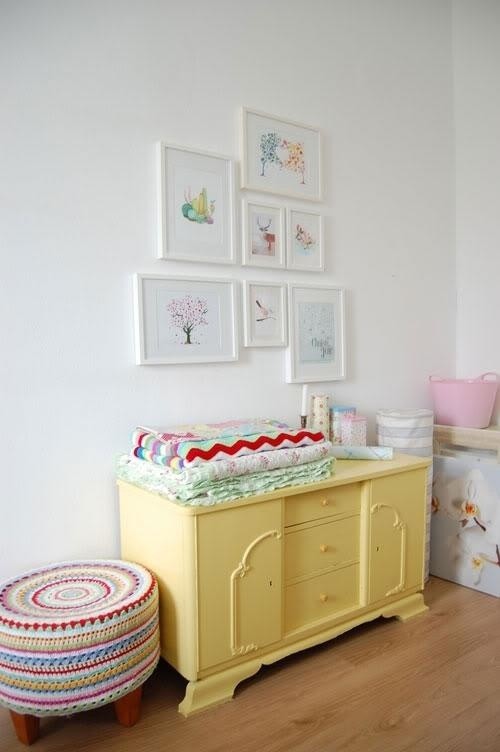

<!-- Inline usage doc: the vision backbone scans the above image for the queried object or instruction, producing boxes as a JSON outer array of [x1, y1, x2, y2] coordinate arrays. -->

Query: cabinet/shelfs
[[432, 421, 500, 467], [116, 448, 438, 717]]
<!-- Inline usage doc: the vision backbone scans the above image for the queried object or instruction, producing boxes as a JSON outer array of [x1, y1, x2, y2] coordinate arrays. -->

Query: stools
[[1, 556, 165, 747]]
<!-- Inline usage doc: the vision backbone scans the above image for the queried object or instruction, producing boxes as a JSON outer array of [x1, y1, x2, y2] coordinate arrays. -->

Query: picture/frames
[[133, 270, 242, 373], [245, 275, 289, 354], [238, 105, 327, 205], [285, 206, 328, 275], [240, 194, 286, 266], [283, 282, 345, 384], [157, 142, 238, 268]]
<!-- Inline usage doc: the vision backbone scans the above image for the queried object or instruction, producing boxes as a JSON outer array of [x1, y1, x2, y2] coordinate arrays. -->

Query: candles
[[299, 383, 308, 418]]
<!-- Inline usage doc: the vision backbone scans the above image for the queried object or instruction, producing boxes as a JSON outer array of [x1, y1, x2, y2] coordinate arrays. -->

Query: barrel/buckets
[[426, 371, 498, 428]]
